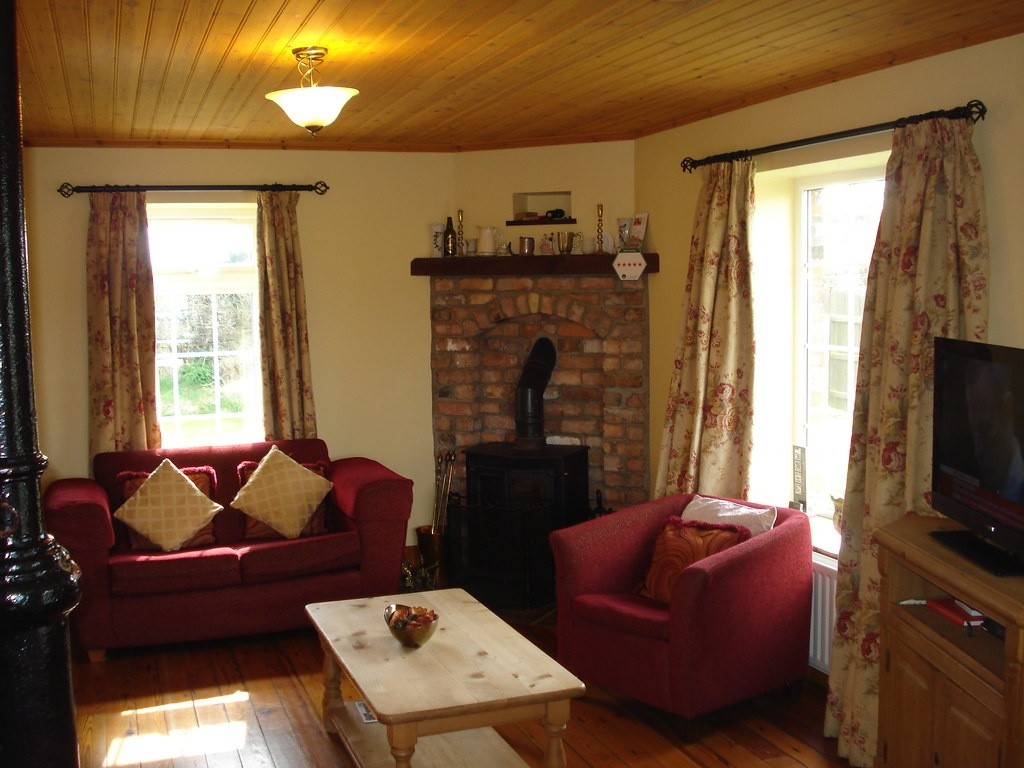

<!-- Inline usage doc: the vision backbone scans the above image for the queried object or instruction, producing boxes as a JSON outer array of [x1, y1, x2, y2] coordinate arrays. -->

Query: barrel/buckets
[[416, 524, 451, 585]]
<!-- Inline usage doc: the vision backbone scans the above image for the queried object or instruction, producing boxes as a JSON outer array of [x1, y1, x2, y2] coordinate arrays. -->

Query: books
[[926, 599, 984, 627]]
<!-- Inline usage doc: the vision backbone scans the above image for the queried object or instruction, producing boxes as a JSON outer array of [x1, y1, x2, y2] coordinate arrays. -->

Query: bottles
[[431, 224, 445, 257], [444, 217, 457, 256]]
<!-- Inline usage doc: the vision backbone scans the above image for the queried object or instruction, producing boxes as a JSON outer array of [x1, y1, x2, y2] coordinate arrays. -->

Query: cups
[[558, 232, 584, 255], [518, 237, 534, 255], [616, 217, 633, 249], [465, 239, 478, 256]]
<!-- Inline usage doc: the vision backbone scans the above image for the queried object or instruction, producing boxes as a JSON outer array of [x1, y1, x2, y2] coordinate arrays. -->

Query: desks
[[305, 588, 586, 768]]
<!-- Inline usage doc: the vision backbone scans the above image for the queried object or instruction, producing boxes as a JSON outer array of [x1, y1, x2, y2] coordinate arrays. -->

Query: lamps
[[265, 46, 359, 138]]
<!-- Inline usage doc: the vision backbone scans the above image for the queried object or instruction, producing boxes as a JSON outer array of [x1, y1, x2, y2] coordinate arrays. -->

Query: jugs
[[477, 225, 504, 255]]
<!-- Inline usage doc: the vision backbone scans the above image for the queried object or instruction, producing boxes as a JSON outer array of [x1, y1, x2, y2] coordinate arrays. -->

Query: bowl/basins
[[384, 604, 440, 650]]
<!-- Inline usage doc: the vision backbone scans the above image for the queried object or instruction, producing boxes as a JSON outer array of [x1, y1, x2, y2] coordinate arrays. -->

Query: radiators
[[809, 552, 838, 676]]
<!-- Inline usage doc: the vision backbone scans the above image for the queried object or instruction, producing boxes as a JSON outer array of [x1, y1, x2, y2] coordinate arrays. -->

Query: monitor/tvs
[[927, 337, 1024, 578]]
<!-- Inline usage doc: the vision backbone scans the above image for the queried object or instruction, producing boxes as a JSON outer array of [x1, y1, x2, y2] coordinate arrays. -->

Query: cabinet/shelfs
[[875, 513, 1024, 768]]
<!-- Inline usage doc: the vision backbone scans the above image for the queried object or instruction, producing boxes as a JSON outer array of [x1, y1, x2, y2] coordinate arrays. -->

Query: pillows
[[116, 466, 217, 549], [238, 461, 328, 541], [113, 457, 225, 552], [680, 494, 777, 538], [635, 514, 751, 607], [230, 445, 335, 538]]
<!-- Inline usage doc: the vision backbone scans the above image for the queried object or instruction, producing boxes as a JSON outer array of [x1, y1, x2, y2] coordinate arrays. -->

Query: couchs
[[43, 439, 414, 661], [549, 497, 813, 719]]
